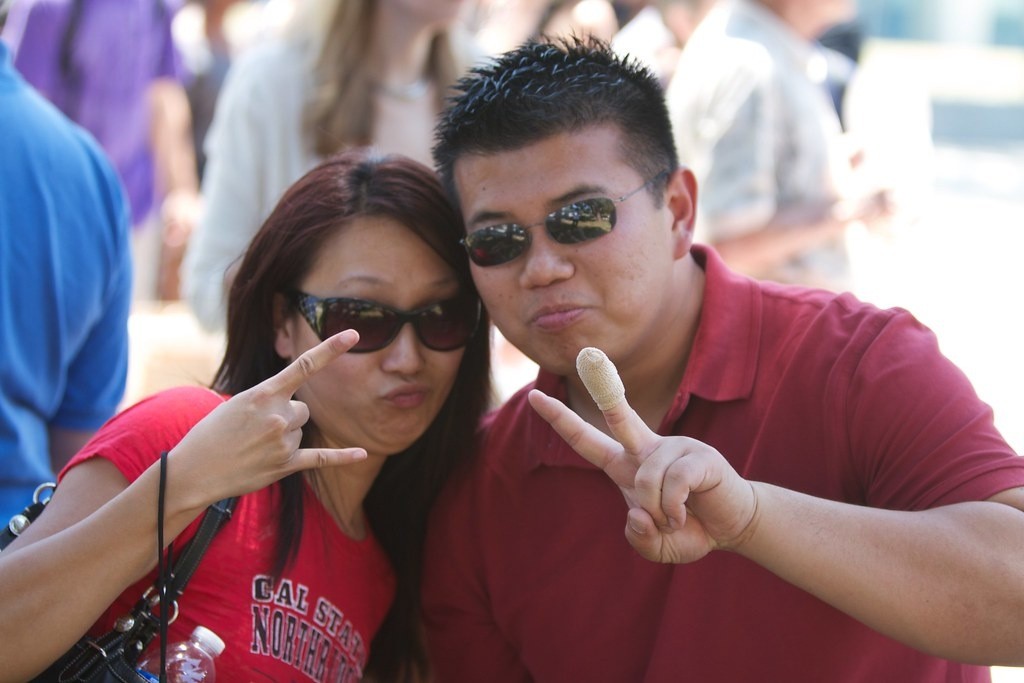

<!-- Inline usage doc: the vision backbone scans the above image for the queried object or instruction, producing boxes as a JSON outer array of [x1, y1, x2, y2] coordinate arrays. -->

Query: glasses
[[286, 288, 482, 353], [459, 167, 673, 266]]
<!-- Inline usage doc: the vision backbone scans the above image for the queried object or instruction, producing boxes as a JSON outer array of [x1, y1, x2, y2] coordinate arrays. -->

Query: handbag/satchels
[[0, 482, 239, 683]]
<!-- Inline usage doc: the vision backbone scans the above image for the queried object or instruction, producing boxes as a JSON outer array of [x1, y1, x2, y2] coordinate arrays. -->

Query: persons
[[0, 0, 133, 547], [372, 30, 1023, 683], [0, 155, 493, 683], [1, 2, 899, 348]]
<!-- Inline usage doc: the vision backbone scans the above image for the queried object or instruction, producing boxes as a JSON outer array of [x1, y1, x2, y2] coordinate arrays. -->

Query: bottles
[[136, 626, 226, 683]]
